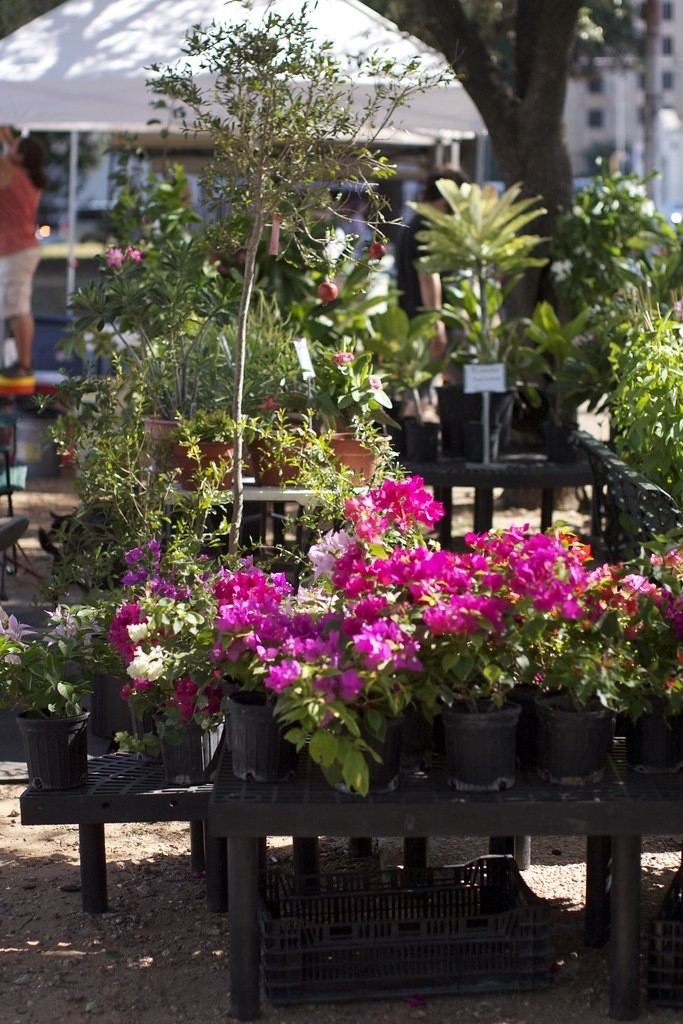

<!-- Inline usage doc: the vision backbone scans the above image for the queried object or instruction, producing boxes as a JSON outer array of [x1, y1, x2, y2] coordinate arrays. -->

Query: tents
[[0, 0, 500, 318]]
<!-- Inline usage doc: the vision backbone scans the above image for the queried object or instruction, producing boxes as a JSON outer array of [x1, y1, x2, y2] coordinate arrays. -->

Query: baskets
[[645, 866, 683, 1009], [258, 856, 553, 1006]]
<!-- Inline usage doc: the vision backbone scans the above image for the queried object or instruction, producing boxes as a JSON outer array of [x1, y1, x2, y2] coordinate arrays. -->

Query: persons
[[0, 121, 47, 382], [394, 168, 472, 433]]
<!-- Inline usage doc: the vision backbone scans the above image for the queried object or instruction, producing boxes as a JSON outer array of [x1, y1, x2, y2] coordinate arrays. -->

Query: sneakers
[[0, 363, 35, 386]]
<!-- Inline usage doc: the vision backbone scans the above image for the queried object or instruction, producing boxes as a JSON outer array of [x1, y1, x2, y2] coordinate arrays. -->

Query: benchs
[[567, 429, 683, 564]]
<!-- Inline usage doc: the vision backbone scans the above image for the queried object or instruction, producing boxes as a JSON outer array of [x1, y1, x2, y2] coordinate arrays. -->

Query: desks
[[398, 441, 608, 553], [20, 749, 683, 1024]]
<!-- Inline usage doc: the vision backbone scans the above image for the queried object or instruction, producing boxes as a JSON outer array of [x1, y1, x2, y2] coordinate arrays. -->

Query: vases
[[324, 432, 392, 486], [442, 699, 522, 793], [435, 380, 515, 460], [144, 414, 181, 480], [533, 691, 617, 787], [621, 695, 683, 773], [246, 435, 303, 484], [228, 691, 280, 784], [335, 716, 403, 796], [173, 440, 234, 493], [16, 708, 91, 791]]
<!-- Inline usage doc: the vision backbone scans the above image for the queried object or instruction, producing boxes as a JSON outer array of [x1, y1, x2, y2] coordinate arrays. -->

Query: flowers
[[0, 242, 683, 796]]
